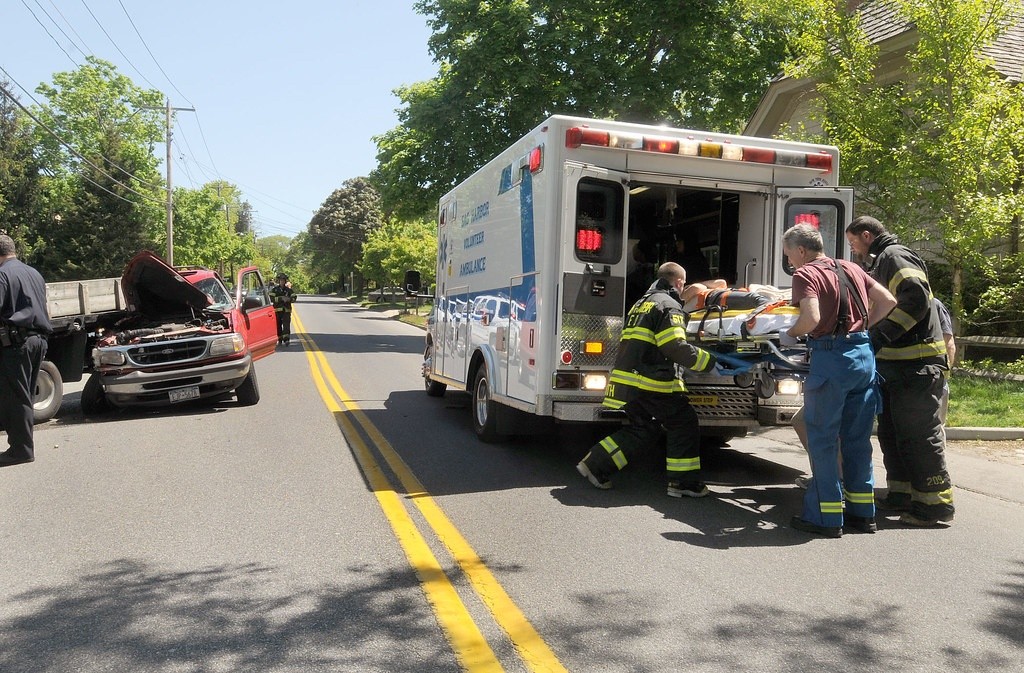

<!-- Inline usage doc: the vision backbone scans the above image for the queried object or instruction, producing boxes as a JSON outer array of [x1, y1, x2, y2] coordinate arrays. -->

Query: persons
[[680, 281, 770, 312], [0, 234, 50, 468], [577, 262, 709, 499], [782, 224, 898, 534], [268, 274, 297, 347], [845, 216, 955, 526], [934, 298, 955, 453]]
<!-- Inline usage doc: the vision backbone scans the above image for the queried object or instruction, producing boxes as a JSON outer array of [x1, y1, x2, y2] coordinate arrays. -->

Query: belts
[[26, 329, 38, 337]]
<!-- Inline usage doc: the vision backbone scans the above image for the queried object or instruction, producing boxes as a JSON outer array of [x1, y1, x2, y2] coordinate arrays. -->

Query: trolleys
[[675, 303, 813, 399]]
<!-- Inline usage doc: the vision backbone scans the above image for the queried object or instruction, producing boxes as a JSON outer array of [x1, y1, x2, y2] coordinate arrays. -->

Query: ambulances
[[401, 114, 856, 446]]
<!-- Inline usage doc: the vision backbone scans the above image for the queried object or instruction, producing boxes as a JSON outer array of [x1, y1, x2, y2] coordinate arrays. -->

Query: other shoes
[[843, 512, 877, 533], [285, 341, 290, 347], [0, 447, 35, 466], [791, 516, 843, 537], [874, 498, 910, 510], [576, 452, 613, 489], [278, 340, 282, 346], [899, 511, 954, 526], [667, 481, 710, 498]]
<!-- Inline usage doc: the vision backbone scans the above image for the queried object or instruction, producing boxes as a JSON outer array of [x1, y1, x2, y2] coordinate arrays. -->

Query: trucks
[[0, 277, 125, 425]]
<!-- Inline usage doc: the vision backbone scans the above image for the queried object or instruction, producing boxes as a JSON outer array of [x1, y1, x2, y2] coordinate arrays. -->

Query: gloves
[[779, 328, 798, 347], [709, 361, 724, 378]]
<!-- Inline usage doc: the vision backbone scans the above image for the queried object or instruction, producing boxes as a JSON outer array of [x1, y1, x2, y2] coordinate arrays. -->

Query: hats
[[276, 273, 287, 280]]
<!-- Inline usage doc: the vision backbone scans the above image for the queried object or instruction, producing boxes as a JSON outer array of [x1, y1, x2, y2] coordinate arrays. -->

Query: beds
[[687, 308, 812, 400]]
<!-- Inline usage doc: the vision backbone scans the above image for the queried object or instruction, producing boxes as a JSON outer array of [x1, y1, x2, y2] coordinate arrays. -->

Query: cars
[[80, 248, 280, 416], [367, 286, 407, 304]]
